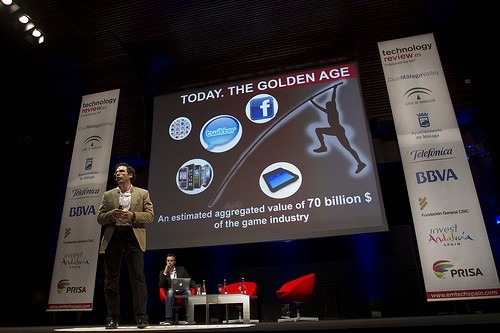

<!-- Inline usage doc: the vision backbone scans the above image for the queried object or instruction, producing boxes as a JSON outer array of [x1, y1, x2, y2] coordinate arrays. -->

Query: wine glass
[[238, 283, 241, 294], [217, 284, 222, 294]]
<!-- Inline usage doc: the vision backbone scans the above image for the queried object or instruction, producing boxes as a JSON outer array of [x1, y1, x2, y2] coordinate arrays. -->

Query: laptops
[[168, 278, 191, 291]]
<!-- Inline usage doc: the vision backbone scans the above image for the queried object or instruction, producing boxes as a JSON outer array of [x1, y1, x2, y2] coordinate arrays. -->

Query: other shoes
[[186, 318, 196, 324], [164, 318, 171, 325]]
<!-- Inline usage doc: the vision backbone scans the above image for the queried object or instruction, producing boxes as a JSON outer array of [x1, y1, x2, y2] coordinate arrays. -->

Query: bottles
[[202, 279, 206, 295], [241, 277, 246, 294], [222, 279, 227, 294]]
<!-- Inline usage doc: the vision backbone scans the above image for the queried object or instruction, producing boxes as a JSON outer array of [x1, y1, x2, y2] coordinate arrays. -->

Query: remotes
[[119, 205, 122, 210]]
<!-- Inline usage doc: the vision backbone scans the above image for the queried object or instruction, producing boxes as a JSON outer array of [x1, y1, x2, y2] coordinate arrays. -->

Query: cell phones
[[178, 163, 211, 190]]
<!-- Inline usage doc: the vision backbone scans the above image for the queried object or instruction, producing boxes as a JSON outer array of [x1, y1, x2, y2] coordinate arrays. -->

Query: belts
[[116, 225, 132, 230]]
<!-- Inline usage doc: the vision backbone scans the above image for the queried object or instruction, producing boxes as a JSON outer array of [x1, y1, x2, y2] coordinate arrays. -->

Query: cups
[[197, 285, 201, 295]]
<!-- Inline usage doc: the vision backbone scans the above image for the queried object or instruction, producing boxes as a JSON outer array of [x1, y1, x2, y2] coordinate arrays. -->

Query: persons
[[96, 163, 155, 329], [158, 254, 197, 326]]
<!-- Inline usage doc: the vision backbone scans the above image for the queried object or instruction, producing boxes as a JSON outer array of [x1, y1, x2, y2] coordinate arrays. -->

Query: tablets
[[262, 168, 300, 192]]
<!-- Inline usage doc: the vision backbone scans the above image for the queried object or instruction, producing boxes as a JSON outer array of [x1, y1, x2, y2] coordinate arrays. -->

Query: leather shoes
[[105, 320, 118, 329], [137, 322, 149, 328]]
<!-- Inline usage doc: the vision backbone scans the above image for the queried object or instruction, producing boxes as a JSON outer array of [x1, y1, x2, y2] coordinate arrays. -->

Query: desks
[[188, 294, 250, 325]]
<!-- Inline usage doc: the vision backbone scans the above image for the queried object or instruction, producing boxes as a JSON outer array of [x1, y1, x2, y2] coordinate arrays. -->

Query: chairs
[[160, 287, 195, 325], [219, 282, 259, 324], [276, 273, 319, 323]]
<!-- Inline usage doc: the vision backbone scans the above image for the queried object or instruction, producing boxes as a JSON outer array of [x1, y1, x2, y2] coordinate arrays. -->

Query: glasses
[[114, 171, 128, 175]]
